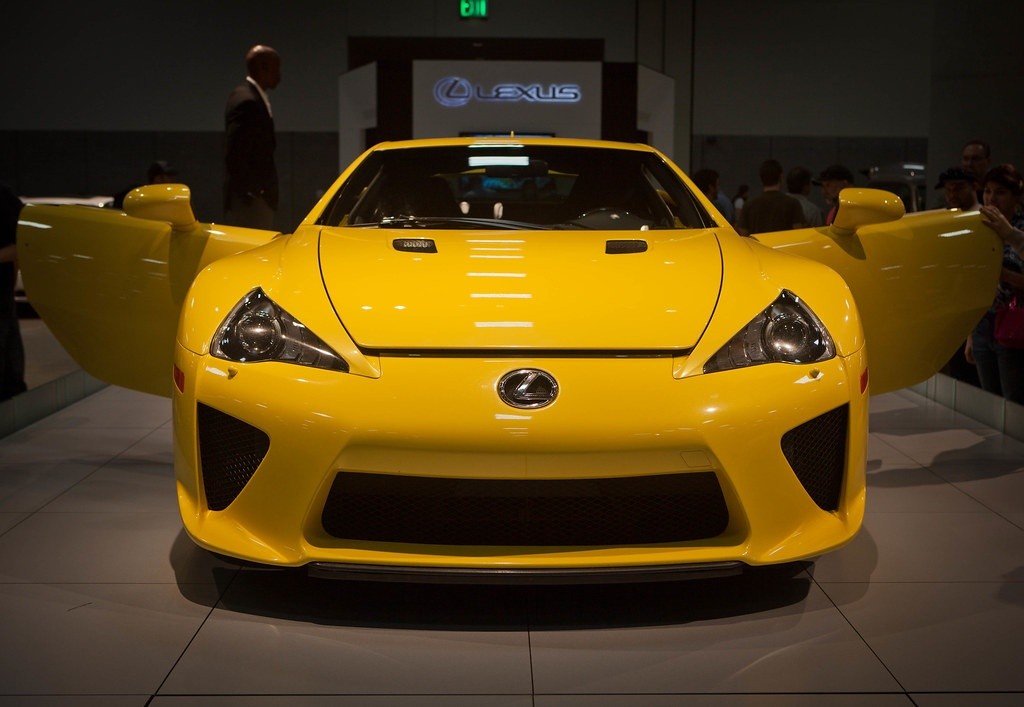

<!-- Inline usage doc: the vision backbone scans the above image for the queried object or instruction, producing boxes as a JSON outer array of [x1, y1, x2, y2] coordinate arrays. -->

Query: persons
[[0, 182, 27, 404], [784, 167, 822, 226], [934, 140, 1024, 406], [811, 166, 854, 226], [594, 169, 649, 218], [731, 185, 749, 220], [736, 159, 806, 236], [223, 45, 283, 231], [716, 191, 733, 221], [146, 163, 173, 185], [696, 169, 720, 201]]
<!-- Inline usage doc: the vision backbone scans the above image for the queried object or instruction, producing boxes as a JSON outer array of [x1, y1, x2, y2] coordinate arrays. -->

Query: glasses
[[960, 155, 985, 162]]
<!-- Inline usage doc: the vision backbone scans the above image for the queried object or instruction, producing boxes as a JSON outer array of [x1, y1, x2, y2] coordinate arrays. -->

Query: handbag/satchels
[[994, 295, 1024, 347]]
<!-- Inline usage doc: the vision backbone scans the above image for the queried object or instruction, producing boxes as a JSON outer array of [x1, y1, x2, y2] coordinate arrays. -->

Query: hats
[[812, 177, 823, 186], [149, 159, 178, 180]]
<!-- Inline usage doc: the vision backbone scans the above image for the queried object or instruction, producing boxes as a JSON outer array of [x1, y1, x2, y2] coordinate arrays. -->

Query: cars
[[17, 128, 1004, 578]]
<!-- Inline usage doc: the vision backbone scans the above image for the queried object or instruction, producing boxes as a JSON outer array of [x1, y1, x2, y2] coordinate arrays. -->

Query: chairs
[[559, 165, 674, 229], [373, 158, 464, 220]]
